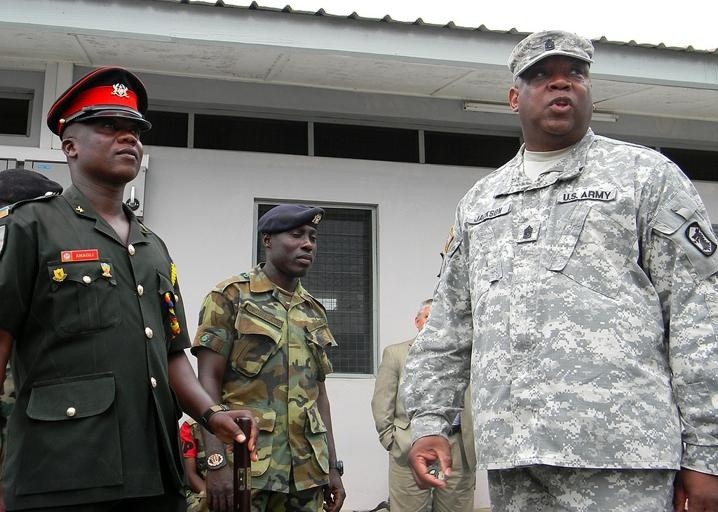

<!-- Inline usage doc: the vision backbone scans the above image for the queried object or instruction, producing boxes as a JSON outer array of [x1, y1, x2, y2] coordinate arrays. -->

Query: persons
[[0, 66, 261, 510], [370, 296, 479, 512], [188, 202, 347, 511], [177, 417, 212, 511], [0, 168, 66, 467], [397, 29, 717, 512]]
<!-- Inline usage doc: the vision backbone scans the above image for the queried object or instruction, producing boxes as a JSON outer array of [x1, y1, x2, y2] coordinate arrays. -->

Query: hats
[[47, 66, 152, 141], [0, 169, 63, 204], [258, 204, 326, 234], [507, 30, 595, 82]]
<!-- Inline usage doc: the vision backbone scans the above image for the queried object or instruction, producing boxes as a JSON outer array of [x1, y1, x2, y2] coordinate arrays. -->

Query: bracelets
[[204, 447, 226, 471], [329, 459, 344, 476], [199, 401, 230, 436]]
